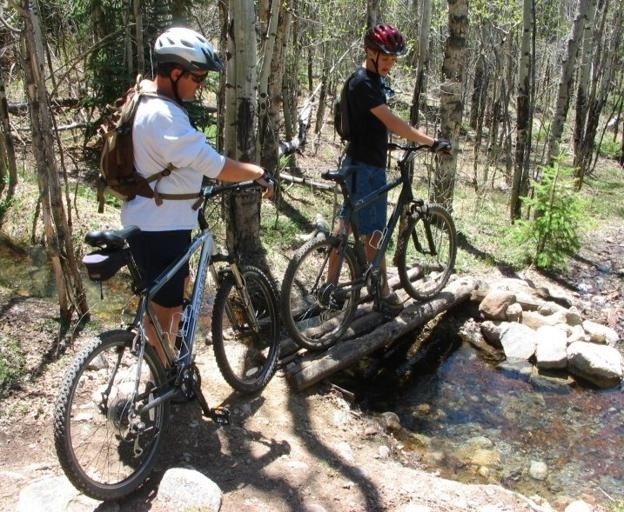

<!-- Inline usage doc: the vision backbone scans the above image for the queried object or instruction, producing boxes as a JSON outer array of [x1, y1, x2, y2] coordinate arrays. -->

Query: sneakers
[[152, 387, 194, 404], [372, 293, 403, 311]]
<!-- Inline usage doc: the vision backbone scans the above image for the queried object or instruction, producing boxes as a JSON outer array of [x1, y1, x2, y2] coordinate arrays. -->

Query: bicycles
[[280, 142, 458, 351], [53, 181, 282, 502]]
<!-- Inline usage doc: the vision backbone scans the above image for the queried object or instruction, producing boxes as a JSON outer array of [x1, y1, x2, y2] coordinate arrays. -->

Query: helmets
[[363, 24, 406, 55], [154, 26, 226, 72]]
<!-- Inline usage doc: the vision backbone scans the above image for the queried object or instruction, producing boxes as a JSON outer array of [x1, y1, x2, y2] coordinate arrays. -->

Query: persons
[[117, 25, 277, 403], [316, 25, 452, 312]]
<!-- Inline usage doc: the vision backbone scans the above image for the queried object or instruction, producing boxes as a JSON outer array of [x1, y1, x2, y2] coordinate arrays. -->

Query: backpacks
[[100, 73, 197, 200], [335, 73, 359, 138]]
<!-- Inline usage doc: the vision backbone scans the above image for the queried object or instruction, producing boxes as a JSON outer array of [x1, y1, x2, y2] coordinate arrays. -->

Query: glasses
[[172, 68, 209, 83]]
